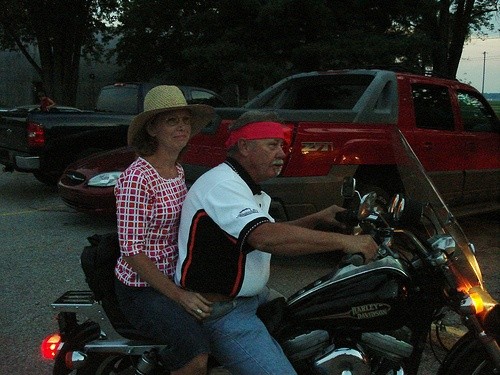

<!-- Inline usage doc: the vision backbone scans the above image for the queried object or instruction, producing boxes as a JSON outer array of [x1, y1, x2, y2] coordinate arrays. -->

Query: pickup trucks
[[0, 83, 232, 197]]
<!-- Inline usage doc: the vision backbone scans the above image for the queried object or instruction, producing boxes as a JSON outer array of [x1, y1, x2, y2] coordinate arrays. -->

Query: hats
[[223, 109, 294, 151], [126, 84, 215, 152]]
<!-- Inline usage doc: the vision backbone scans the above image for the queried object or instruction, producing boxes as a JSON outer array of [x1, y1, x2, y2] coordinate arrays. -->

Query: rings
[[197, 308, 201, 315]]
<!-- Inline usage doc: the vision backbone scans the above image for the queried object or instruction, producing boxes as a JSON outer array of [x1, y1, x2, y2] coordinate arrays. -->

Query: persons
[[175, 110, 379, 375], [115, 85, 213, 375], [36, 90, 55, 112]]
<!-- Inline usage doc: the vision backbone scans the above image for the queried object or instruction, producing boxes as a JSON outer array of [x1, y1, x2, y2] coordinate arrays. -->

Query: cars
[[58, 69, 500, 264]]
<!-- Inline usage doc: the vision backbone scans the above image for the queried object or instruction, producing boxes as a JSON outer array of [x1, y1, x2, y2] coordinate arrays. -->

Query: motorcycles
[[41, 174, 500, 374]]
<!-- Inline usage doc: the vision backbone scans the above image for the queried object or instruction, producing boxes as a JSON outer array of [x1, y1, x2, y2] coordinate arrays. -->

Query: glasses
[[166, 112, 194, 127]]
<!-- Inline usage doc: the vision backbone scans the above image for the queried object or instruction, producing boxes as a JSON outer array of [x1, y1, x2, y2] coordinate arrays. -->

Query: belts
[[197, 291, 232, 302]]
